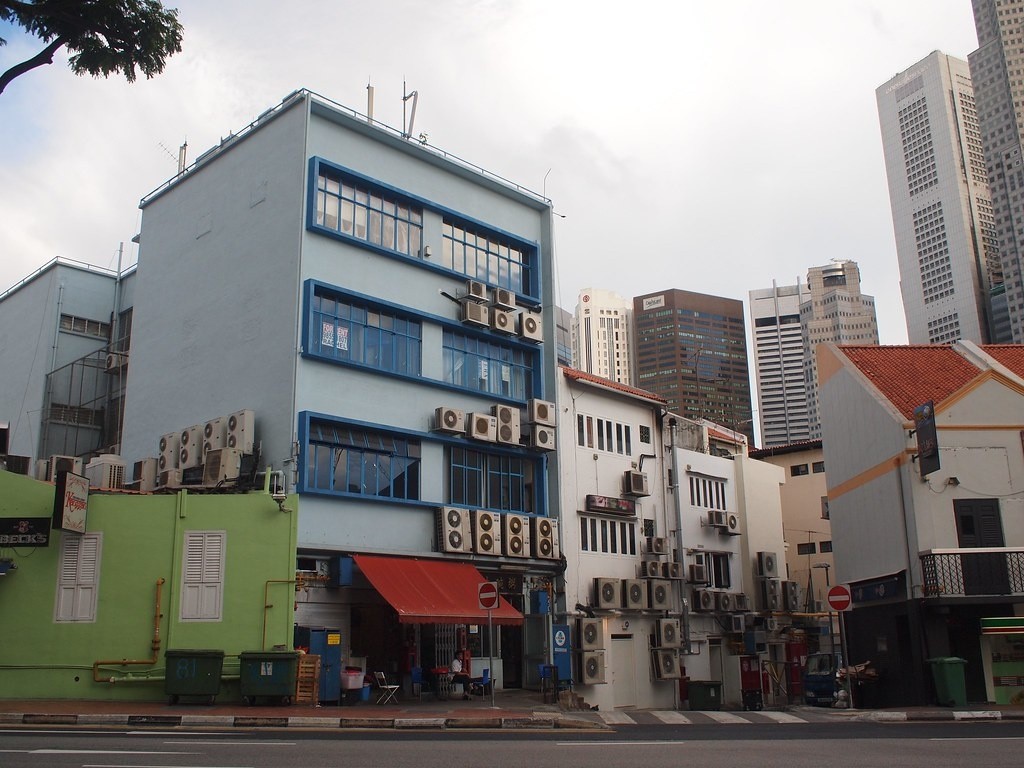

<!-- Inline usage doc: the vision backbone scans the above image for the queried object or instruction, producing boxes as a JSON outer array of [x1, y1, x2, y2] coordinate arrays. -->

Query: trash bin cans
[[926, 657, 968, 708], [164, 649, 225, 705], [687, 680, 723, 711], [239, 649, 300, 706]]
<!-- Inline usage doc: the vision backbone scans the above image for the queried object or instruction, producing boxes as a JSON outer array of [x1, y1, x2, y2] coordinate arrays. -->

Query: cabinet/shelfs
[[309, 631, 343, 704]]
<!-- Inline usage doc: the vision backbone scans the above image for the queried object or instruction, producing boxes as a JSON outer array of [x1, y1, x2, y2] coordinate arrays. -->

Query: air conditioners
[[35, 445, 124, 489], [783, 581, 798, 611], [435, 397, 557, 449], [761, 581, 781, 611], [756, 552, 778, 577], [131, 410, 255, 491], [435, 506, 559, 560], [726, 612, 769, 654], [624, 471, 649, 495], [721, 511, 741, 535], [708, 511, 727, 525], [105, 351, 119, 368], [459, 281, 542, 342], [689, 564, 707, 582], [733, 593, 750, 611], [693, 590, 715, 611], [574, 538, 682, 683], [715, 592, 733, 611]]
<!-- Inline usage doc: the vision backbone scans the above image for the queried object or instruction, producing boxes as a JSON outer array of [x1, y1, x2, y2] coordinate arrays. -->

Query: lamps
[[501, 565, 530, 571]]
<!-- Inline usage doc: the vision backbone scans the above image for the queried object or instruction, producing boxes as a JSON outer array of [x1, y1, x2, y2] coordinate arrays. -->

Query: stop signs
[[826, 584, 853, 612], [477, 582, 498, 610]]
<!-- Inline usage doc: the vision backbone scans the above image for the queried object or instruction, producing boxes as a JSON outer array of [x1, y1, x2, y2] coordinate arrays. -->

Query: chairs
[[375, 672, 399, 704], [468, 668, 490, 702], [538, 665, 555, 693], [408, 667, 430, 702]]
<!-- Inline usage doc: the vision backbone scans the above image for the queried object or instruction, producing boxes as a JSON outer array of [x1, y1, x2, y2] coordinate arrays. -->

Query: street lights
[[814, 562, 839, 694]]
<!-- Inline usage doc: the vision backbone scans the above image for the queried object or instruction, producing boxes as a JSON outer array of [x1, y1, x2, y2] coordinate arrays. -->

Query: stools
[[448, 682, 464, 698], [432, 667, 451, 696]]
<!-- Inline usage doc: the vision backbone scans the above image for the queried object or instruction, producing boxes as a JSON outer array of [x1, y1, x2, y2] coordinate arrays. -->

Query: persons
[[447, 651, 479, 700]]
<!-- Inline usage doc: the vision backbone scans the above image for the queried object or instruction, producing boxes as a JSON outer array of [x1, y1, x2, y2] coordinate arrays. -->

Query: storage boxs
[[401, 644, 418, 673], [341, 671, 372, 702]]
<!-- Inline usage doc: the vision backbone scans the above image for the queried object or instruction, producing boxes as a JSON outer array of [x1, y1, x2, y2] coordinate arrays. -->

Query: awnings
[[351, 552, 524, 627]]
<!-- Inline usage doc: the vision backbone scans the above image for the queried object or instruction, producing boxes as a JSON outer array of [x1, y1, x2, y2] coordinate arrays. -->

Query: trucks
[[799, 652, 844, 705]]
